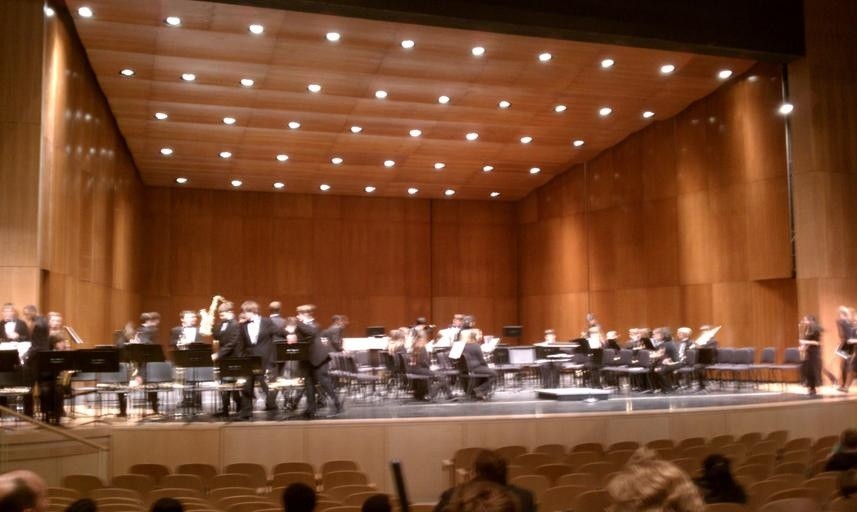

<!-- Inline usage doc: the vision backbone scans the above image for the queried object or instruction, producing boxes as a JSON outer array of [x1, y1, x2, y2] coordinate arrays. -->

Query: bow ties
[[248, 320, 254, 323]]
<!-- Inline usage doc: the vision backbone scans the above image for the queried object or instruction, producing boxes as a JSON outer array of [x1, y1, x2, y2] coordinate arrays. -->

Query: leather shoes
[[447, 393, 457, 401], [44, 418, 60, 425], [152, 398, 157, 412], [118, 412, 127, 417], [632, 387, 670, 394], [283, 401, 340, 414], [474, 387, 488, 401], [213, 398, 241, 417], [833, 384, 848, 393], [265, 402, 278, 411], [424, 394, 437, 403]]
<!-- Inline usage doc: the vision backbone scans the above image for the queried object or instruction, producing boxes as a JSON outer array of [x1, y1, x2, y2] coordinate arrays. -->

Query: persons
[[0, 303, 70, 424], [835, 304, 857, 392], [432, 449, 536, 510], [115, 312, 161, 418], [826, 429, 857, 511], [1, 470, 185, 511], [608, 452, 747, 511], [385, 314, 497, 402], [170, 300, 350, 417], [581, 314, 719, 394], [281, 483, 395, 512], [797, 312, 821, 399]]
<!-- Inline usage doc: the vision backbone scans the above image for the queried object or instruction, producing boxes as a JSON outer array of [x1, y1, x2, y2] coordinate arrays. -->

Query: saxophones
[[199, 296, 227, 336]]
